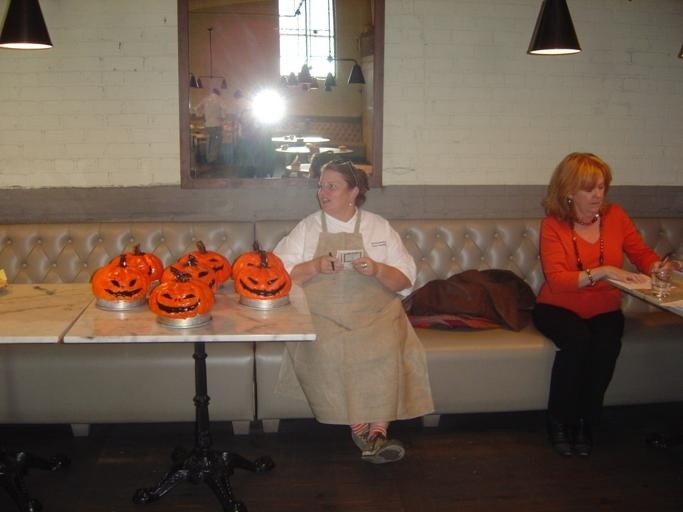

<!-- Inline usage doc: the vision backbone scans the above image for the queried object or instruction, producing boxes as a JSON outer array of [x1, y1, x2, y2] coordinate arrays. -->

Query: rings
[[361, 262, 367, 268]]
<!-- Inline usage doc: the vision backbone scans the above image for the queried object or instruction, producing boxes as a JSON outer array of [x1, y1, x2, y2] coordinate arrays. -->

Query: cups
[[650, 268, 673, 298]]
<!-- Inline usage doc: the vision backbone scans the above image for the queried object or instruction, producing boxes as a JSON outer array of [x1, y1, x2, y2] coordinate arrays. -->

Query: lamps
[[186, 10, 229, 90], [0, 0, 53, 50], [526, 0, 581, 57], [279, 0, 365, 92]]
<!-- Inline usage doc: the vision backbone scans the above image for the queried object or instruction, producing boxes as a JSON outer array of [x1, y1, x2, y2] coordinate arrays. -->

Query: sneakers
[[351, 430, 405, 465], [549, 424, 592, 463]]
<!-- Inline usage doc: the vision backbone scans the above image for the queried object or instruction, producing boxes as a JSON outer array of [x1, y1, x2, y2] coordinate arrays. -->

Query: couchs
[[0, 219, 682, 441]]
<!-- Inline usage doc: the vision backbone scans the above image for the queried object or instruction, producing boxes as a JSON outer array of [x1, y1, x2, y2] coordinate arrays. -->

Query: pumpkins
[[160, 255, 219, 294], [179, 240, 232, 282], [149, 272, 215, 318], [235, 255, 291, 298], [91, 255, 150, 301], [230, 241, 284, 282], [109, 243, 163, 283]]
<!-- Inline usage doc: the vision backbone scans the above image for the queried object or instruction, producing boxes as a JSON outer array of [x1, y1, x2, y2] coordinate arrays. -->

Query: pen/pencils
[[329, 252, 334, 272], [659, 251, 673, 269]]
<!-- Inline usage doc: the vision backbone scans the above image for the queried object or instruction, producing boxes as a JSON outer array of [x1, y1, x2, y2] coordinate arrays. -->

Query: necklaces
[[571, 211, 604, 271]]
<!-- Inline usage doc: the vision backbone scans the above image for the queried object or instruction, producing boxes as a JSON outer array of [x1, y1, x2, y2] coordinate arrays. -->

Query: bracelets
[[587, 268, 595, 287]]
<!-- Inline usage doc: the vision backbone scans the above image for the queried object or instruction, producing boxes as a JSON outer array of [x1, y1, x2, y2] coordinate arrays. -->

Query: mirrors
[[176, 0, 385, 189]]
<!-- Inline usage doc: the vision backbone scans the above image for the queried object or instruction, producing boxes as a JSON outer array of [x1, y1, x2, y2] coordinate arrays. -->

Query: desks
[[1, 280, 316, 510], [270, 134, 372, 177]]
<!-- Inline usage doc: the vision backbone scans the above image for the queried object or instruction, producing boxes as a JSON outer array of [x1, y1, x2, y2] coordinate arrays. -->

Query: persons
[[194, 88, 224, 166], [534, 152, 674, 457], [272, 160, 417, 464]]
